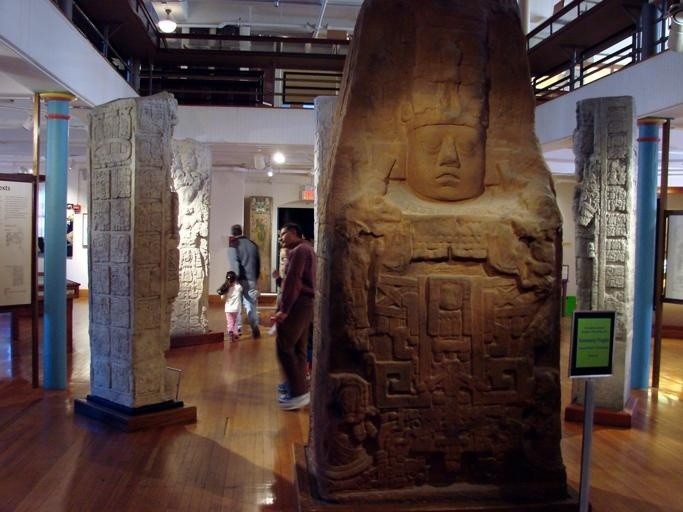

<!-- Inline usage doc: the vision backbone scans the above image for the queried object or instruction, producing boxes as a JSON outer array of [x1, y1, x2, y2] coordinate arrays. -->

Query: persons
[[395, 47, 492, 199], [215, 272, 246, 342], [229, 224, 262, 342], [271, 269, 314, 382], [270, 222, 316, 413]]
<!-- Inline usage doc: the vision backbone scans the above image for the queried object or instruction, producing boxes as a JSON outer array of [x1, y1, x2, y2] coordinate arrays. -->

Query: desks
[[8, 271, 82, 354]]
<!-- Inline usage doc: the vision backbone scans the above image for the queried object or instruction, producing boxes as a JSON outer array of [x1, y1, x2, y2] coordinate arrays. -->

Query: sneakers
[[276, 371, 311, 411], [228, 326, 259, 342]]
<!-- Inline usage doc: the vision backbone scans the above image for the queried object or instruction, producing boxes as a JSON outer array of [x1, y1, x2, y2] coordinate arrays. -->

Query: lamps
[[254, 154, 272, 171]]
